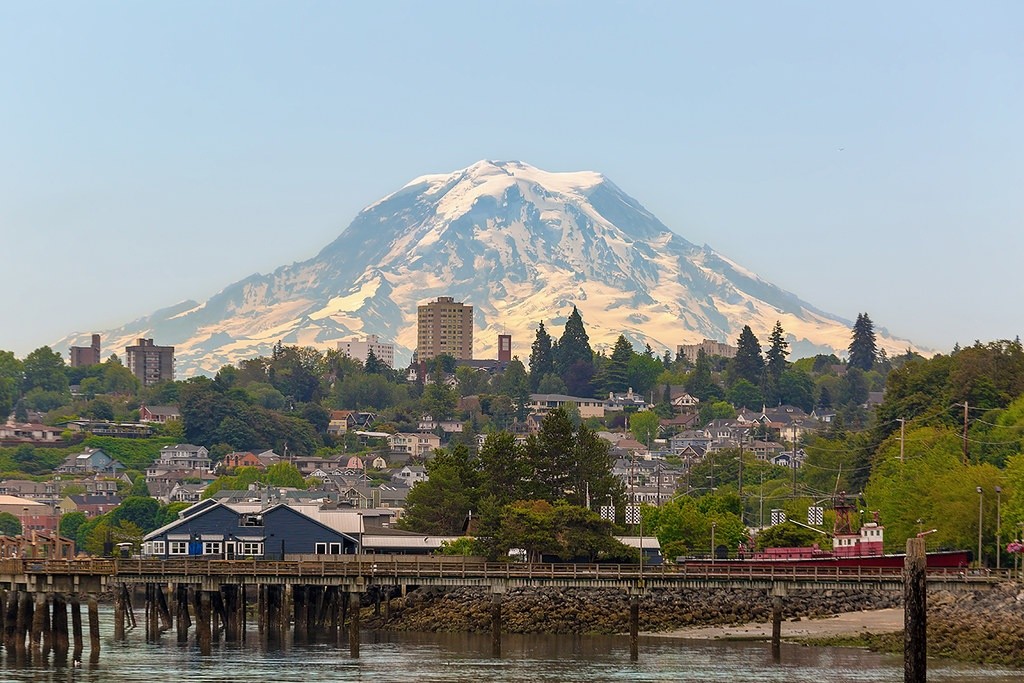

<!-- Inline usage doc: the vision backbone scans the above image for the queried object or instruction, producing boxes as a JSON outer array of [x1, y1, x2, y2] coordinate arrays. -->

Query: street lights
[[23, 508, 28, 556], [995, 485, 1003, 568], [54, 505, 60, 560], [977, 486, 983, 568], [710, 521, 716, 564], [357, 512, 364, 577]]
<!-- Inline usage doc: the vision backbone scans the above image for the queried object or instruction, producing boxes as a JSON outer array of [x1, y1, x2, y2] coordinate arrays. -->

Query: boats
[[676, 490, 973, 575]]
